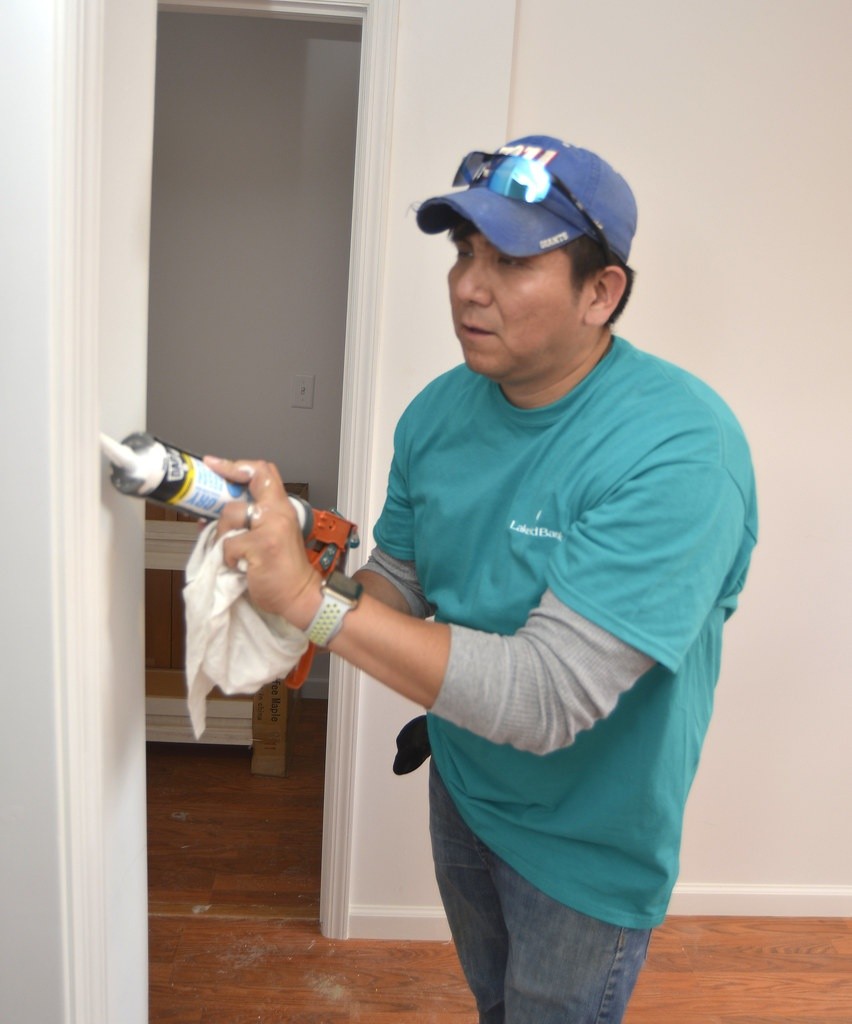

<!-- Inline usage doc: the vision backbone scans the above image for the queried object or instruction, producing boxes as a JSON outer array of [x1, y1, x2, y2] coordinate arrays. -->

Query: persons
[[205, 136, 759, 1024]]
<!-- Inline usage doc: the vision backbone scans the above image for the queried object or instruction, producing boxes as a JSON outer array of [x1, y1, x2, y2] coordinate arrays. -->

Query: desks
[[144, 483, 311, 776]]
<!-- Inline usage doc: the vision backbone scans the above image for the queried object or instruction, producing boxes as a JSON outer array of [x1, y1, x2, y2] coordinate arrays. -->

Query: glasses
[[452, 151, 618, 264]]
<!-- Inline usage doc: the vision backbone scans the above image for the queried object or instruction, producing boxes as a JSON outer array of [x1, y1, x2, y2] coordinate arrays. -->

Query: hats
[[416, 135, 637, 264]]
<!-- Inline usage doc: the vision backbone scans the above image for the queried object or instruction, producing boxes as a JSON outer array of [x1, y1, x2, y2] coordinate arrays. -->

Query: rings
[[242, 501, 261, 528]]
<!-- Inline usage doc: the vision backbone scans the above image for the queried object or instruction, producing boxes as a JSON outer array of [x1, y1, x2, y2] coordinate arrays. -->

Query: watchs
[[301, 570, 363, 649]]
